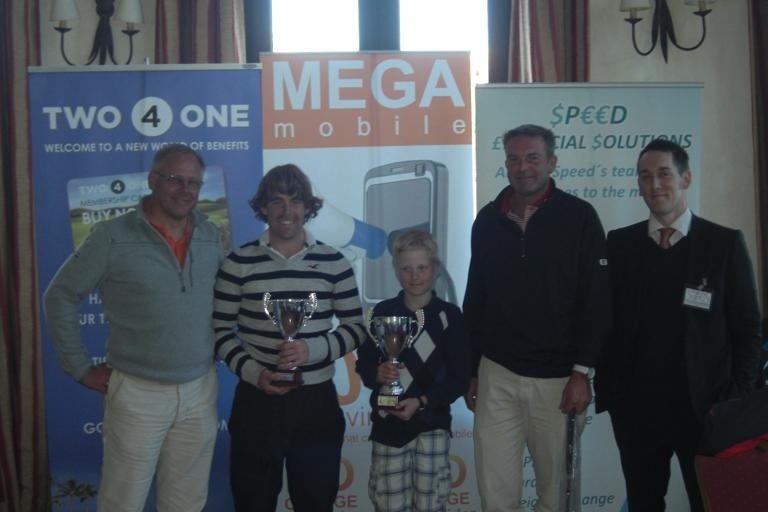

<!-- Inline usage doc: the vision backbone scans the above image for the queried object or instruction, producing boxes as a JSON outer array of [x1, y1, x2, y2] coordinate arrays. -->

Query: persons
[[355, 227, 469, 512], [41, 144, 225, 511], [461, 122, 606, 512], [213, 162, 364, 512], [591, 140, 767, 509]]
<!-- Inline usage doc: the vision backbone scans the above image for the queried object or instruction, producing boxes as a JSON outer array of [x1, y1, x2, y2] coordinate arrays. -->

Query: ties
[[658, 229, 677, 249]]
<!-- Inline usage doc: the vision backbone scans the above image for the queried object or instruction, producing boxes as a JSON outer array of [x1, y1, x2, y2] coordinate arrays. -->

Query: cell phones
[[362, 160, 448, 315]]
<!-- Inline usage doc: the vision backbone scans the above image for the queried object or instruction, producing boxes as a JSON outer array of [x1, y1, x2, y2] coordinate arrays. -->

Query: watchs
[[573, 363, 596, 379], [418, 396, 426, 412]]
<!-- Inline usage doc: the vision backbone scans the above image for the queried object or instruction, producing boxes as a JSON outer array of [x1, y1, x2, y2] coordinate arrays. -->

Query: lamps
[[618, 0, 716, 65], [47, 0, 144, 67]]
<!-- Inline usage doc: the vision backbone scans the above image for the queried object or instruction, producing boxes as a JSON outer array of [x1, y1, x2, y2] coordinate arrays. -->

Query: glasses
[[152, 170, 204, 190]]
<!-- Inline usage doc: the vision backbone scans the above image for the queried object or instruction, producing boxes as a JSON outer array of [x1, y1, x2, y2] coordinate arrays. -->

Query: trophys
[[263, 291, 318, 386], [366, 305, 426, 410]]
